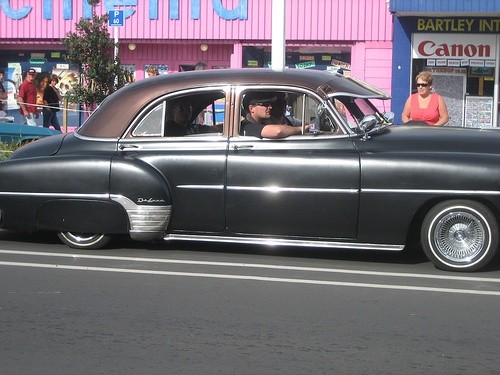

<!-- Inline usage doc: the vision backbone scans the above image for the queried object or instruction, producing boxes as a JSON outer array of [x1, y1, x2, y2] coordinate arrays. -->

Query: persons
[[146, 67, 159, 77], [164, 99, 223, 137], [43, 75, 60, 131], [401, 71, 449, 126], [0, 70, 8, 117], [17, 69, 39, 126], [36, 72, 50, 106], [271, 96, 311, 127], [240, 94, 310, 138]]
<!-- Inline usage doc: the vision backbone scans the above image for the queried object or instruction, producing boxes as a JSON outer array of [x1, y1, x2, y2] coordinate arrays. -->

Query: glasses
[[254, 102, 272, 106], [416, 83, 429, 87]]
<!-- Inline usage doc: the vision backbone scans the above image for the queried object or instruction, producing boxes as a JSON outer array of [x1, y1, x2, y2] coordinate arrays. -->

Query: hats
[[243, 92, 276, 104], [27, 69, 36, 75]]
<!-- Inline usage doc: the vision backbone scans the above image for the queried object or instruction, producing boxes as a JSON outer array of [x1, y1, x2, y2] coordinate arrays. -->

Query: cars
[[0, 68, 500, 273]]
